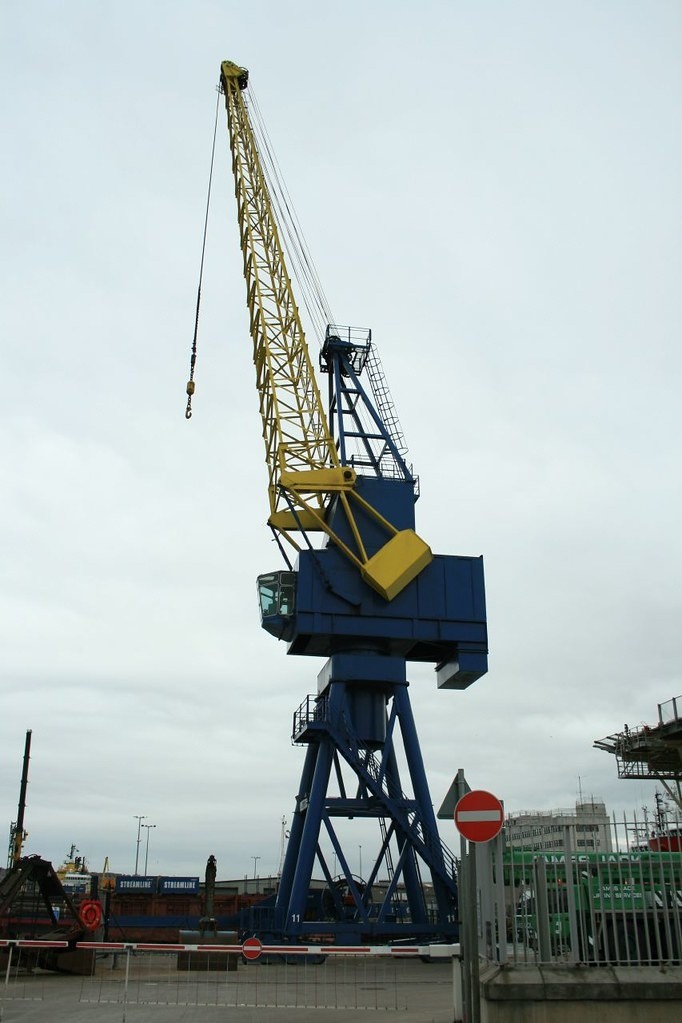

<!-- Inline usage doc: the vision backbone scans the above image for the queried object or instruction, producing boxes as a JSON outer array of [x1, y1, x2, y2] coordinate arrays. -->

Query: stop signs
[[242, 937, 264, 961], [454, 789, 505, 845]]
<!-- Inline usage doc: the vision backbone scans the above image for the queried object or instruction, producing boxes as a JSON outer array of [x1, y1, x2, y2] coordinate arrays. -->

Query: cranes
[[182, 58, 487, 965]]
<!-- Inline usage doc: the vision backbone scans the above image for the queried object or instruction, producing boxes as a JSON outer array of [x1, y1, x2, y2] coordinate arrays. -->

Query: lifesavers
[[82, 904, 100, 929]]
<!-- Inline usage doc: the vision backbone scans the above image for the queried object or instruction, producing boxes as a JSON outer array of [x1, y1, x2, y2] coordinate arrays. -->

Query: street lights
[[141, 824, 156, 876], [333, 852, 338, 882], [133, 815, 147, 876], [250, 856, 260, 880], [359, 845, 363, 884]]
